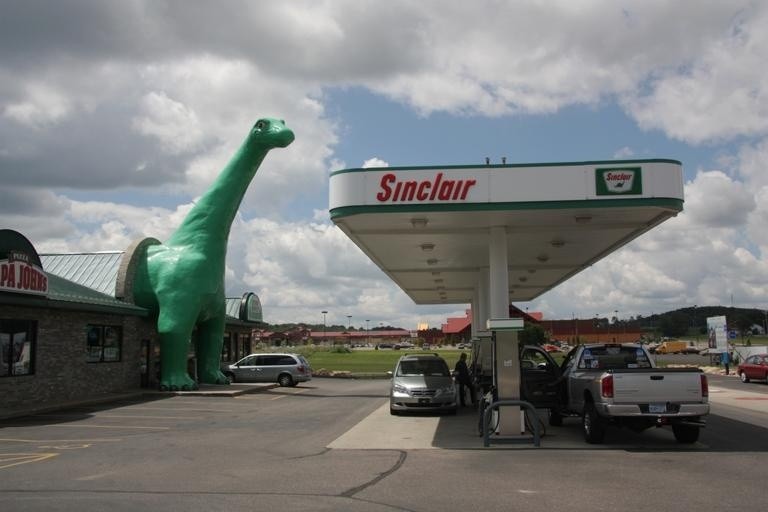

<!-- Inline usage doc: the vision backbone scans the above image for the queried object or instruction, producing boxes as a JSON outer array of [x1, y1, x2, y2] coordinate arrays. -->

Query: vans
[[223, 352, 312, 386]]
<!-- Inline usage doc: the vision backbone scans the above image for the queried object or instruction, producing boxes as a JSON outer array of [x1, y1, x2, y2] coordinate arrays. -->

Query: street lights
[[693, 304, 700, 347], [320, 310, 331, 350], [365, 319, 371, 345], [614, 309, 620, 342], [346, 315, 354, 346], [595, 313, 602, 344]]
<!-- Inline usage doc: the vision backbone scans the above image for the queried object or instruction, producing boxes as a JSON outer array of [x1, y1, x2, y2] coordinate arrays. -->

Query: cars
[[644, 341, 728, 356], [386, 352, 464, 414], [337, 340, 377, 349], [422, 342, 431, 351], [379, 343, 397, 348], [458, 342, 465, 349], [736, 352, 768, 383]]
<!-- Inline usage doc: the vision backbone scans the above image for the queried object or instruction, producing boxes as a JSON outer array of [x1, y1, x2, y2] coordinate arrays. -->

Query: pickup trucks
[[520, 341, 714, 445], [395, 341, 415, 348]]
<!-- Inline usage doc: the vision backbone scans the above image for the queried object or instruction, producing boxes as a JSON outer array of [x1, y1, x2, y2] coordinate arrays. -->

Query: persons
[[547, 367, 574, 404], [454, 352, 474, 407]]
